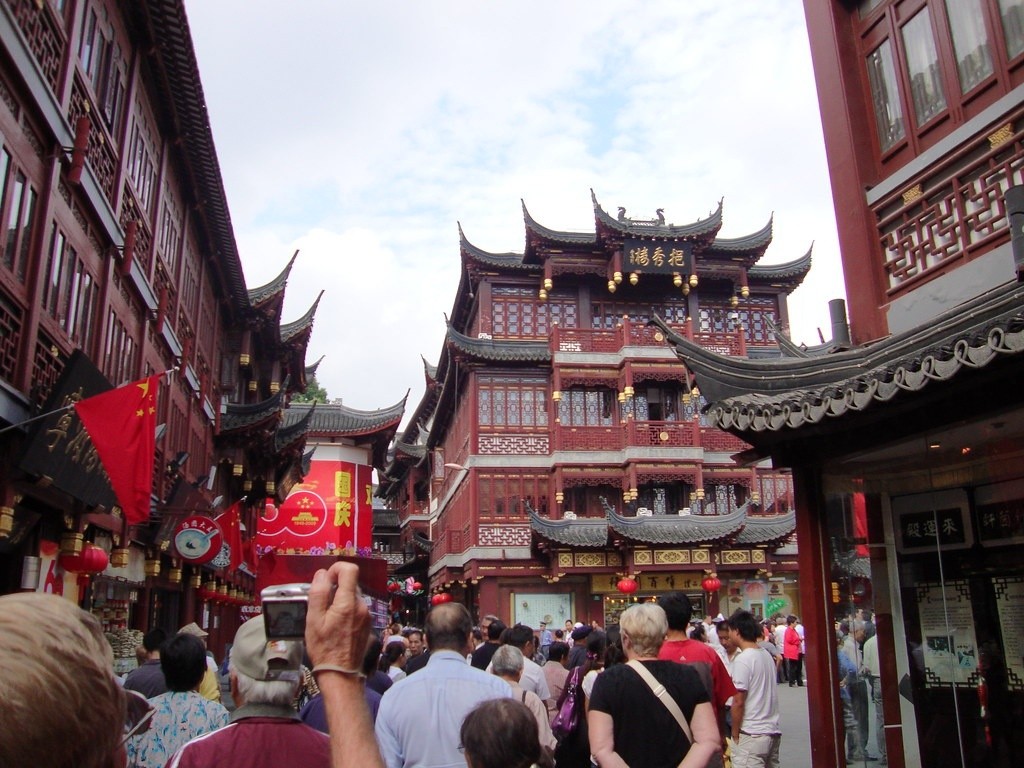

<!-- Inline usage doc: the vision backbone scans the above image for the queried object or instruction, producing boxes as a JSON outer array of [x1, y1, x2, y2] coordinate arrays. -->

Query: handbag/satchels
[[551, 664, 580, 741], [719, 736, 733, 768], [850, 680, 866, 699]]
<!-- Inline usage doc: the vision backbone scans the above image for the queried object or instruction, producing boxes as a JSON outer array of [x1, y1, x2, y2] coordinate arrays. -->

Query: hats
[[573, 622, 583, 630], [712, 618, 722, 624], [690, 618, 697, 624], [540, 621, 546, 625], [175, 622, 209, 639], [572, 626, 592, 639], [695, 618, 702, 624], [229, 614, 303, 681]]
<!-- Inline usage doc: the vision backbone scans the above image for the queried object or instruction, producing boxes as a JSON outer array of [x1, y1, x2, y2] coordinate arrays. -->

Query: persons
[[379, 542, 383, 553], [373, 602, 514, 768], [587, 603, 723, 768], [124, 614, 627, 768], [457, 698, 540, 768], [374, 539, 378, 554], [654, 591, 739, 768], [0, 561, 386, 768], [686, 608, 888, 768]]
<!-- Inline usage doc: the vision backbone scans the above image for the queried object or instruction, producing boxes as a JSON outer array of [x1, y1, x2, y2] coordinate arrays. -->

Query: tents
[[701, 573, 722, 603]]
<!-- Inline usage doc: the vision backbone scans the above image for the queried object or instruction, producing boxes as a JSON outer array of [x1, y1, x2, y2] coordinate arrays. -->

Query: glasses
[[457, 742, 466, 754], [111, 690, 157, 752]]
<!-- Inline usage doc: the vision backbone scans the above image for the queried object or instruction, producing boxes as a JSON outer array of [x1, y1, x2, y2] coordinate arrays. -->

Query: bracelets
[[310, 664, 367, 690]]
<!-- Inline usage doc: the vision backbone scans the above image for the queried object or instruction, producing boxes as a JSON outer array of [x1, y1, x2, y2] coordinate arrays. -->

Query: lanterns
[[412, 580, 424, 593], [616, 575, 638, 603], [57, 541, 110, 587], [198, 582, 261, 607], [388, 577, 404, 596], [432, 593, 451, 609]]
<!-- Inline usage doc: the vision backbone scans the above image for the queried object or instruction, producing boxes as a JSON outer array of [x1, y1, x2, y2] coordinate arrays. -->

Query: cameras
[[261, 583, 339, 641]]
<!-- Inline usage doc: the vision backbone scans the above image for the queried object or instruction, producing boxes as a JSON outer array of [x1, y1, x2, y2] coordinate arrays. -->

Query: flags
[[75, 372, 164, 525], [216, 502, 244, 572]]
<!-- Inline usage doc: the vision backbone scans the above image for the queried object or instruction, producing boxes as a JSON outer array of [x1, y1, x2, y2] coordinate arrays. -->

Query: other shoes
[[880, 753, 887, 765], [845, 759, 854, 764], [867, 755, 878, 761]]
